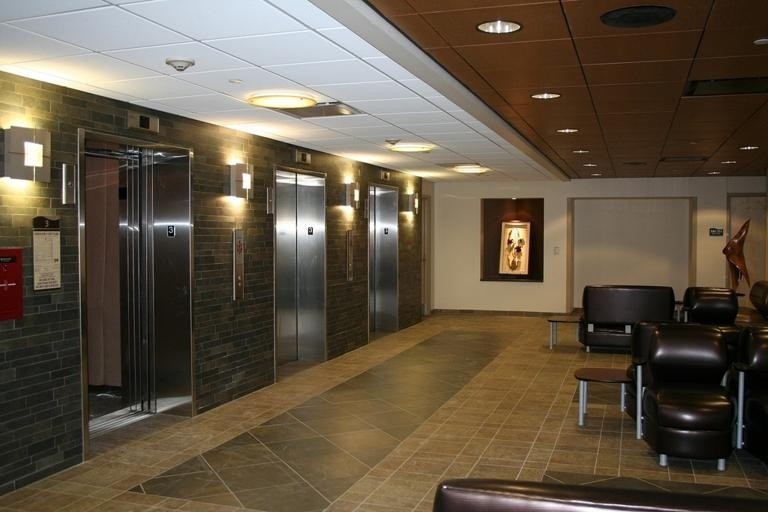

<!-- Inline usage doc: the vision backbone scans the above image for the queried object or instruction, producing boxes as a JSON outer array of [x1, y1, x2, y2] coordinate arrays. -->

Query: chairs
[[626, 279, 767, 470]]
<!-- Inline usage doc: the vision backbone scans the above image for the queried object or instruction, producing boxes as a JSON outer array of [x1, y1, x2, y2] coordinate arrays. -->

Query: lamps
[[231, 163, 254, 200], [0, 125, 51, 184], [346, 182, 360, 210], [408, 192, 418, 215]]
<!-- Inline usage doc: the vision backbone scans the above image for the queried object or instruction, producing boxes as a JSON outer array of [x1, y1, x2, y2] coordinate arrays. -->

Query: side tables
[[574, 368, 632, 427], [548, 316, 581, 352]]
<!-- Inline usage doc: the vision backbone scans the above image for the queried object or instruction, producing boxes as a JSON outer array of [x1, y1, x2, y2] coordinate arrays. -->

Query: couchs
[[578, 285, 675, 353], [433, 478, 768, 512]]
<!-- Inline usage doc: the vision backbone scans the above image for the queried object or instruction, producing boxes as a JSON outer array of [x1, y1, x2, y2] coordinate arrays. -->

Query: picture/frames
[[499, 222, 530, 275]]
